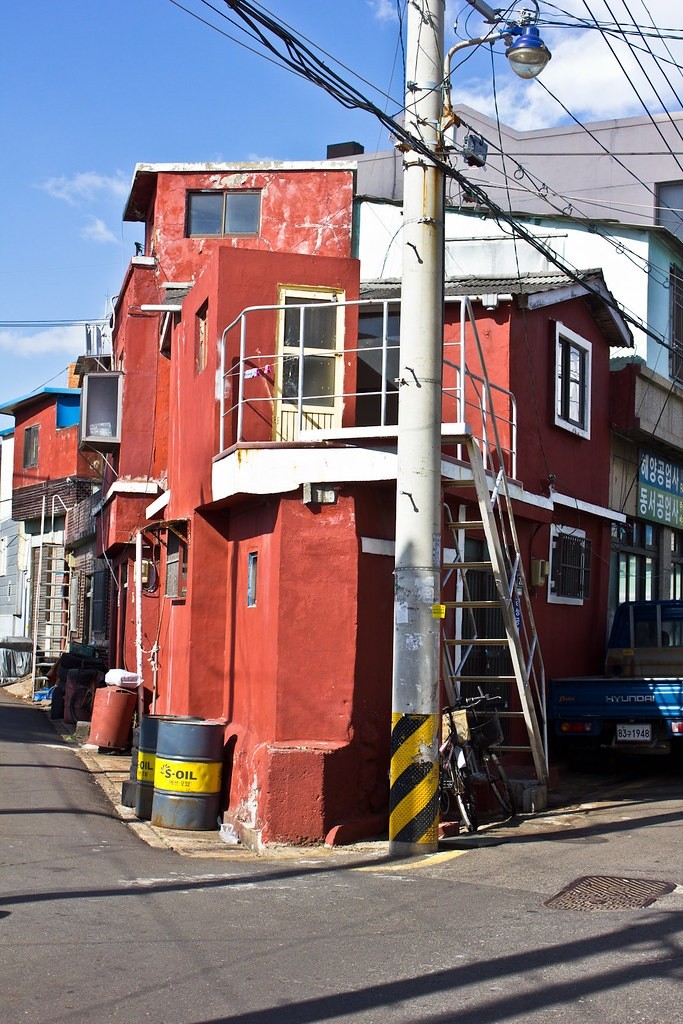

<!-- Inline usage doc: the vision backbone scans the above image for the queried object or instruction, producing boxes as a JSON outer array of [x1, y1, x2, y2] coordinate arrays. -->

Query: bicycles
[[439, 685, 516, 833], [68, 668, 95, 723]]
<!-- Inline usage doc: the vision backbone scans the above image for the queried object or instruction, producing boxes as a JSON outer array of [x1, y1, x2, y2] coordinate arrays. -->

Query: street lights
[[439, 17, 551, 149]]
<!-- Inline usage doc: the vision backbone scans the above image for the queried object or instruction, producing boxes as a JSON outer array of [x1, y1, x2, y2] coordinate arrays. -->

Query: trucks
[[539, 599, 683, 781]]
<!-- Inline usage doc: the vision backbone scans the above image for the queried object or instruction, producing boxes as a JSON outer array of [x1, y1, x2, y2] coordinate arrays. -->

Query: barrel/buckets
[[151, 720, 225, 830], [135, 717, 204, 821]]
